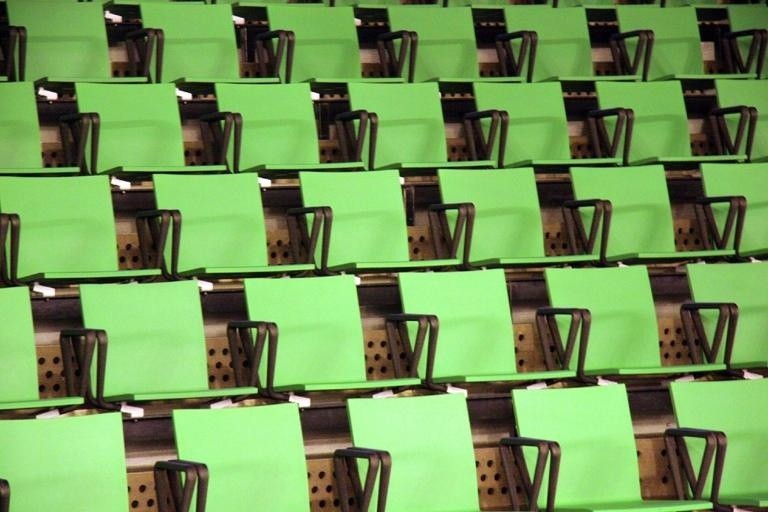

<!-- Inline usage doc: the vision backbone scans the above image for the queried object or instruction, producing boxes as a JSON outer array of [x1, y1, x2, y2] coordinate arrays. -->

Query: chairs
[[0, 0, 767, 511]]
